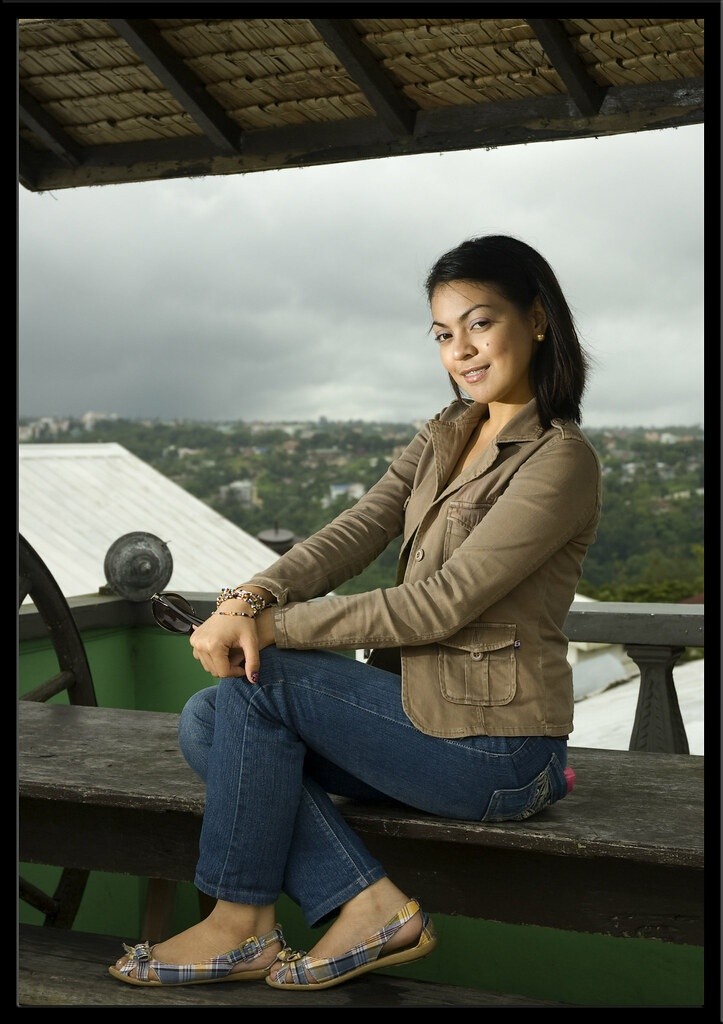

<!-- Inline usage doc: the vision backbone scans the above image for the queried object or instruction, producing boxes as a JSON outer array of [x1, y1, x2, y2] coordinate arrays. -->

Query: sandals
[[266, 898, 436, 989], [109, 923, 285, 985]]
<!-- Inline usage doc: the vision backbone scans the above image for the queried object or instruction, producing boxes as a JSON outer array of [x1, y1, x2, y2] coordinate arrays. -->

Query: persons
[[107, 234, 601, 989]]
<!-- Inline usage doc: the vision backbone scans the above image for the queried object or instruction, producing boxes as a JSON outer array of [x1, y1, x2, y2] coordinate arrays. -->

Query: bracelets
[[212, 589, 266, 619]]
